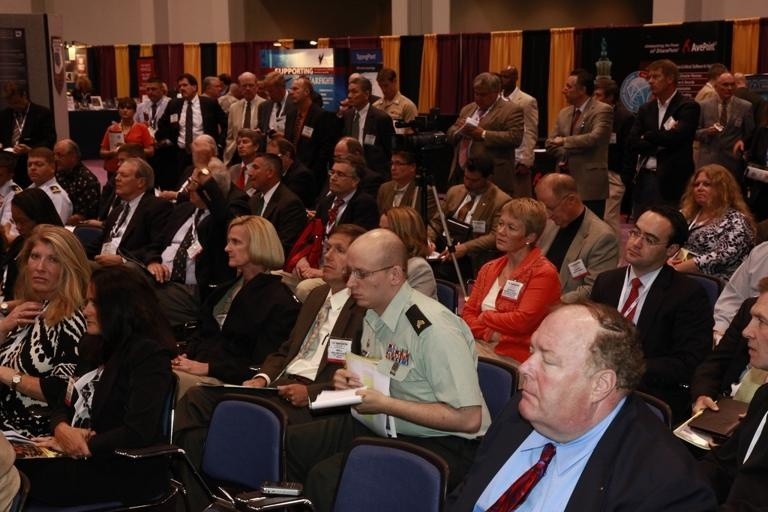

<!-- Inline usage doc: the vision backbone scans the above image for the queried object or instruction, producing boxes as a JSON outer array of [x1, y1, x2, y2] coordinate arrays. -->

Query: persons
[[1, 58, 767, 512]]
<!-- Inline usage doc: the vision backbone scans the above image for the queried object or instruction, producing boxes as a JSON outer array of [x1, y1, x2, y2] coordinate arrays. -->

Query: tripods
[[410, 154, 468, 298]]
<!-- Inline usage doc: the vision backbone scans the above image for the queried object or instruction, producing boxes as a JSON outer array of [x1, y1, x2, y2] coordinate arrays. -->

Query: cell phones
[[259, 479, 304, 497]]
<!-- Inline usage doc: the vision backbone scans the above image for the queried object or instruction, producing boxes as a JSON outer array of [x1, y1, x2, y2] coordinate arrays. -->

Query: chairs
[[684, 271, 724, 310], [115, 392, 289, 511], [477, 355, 519, 425], [434, 277, 460, 313], [635, 389, 674, 433], [247, 436, 452, 512]]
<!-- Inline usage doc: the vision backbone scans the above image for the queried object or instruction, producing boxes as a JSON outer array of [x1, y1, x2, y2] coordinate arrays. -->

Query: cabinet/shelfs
[[67, 109, 122, 163]]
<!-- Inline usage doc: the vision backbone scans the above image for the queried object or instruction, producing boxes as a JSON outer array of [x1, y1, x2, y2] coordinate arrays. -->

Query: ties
[[328, 198, 345, 226], [459, 137, 470, 168], [170, 209, 205, 284], [243, 101, 252, 128], [185, 102, 193, 151], [570, 110, 580, 134], [457, 193, 477, 221], [236, 166, 247, 190], [256, 194, 265, 216], [294, 113, 302, 144], [487, 443, 555, 511], [352, 112, 360, 139], [275, 102, 282, 118], [620, 278, 641, 320], [297, 298, 331, 361], [720, 102, 727, 128], [111, 203, 129, 239], [151, 104, 157, 129]]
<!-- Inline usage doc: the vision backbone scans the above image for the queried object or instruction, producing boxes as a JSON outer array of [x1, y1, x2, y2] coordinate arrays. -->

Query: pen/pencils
[[84, 427, 92, 461], [343, 364, 350, 383]]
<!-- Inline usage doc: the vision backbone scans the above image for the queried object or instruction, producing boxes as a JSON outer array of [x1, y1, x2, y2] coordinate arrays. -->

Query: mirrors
[[59, 43, 93, 112]]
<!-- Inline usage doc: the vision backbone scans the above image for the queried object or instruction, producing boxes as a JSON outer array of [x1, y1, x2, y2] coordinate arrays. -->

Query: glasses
[[342, 265, 396, 280], [628, 230, 667, 247], [389, 162, 408, 167], [328, 170, 356, 180], [187, 176, 200, 190]]
